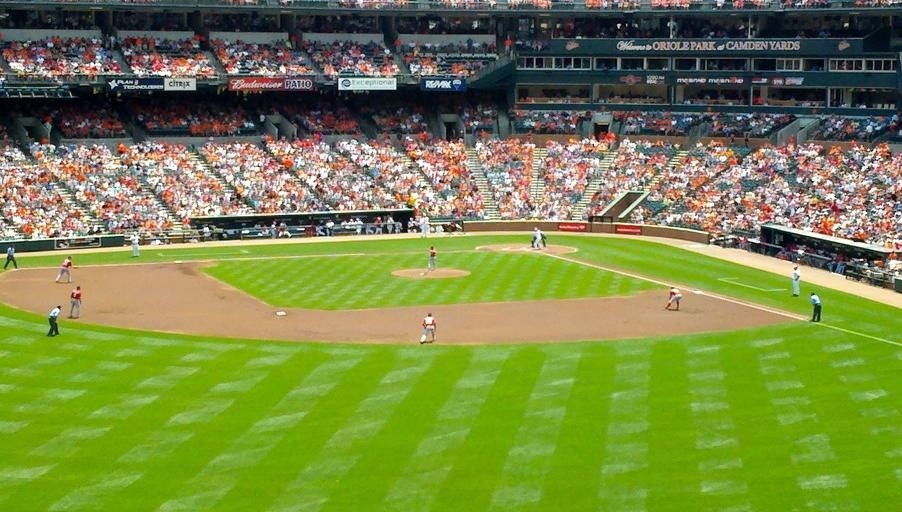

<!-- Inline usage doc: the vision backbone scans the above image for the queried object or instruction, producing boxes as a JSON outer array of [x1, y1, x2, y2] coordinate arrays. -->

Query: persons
[[47, 305, 62, 336], [419, 313, 437, 343], [665, 286, 682, 311], [809, 292, 822, 322], [791, 265, 800, 296], [69, 286, 82, 318]]
